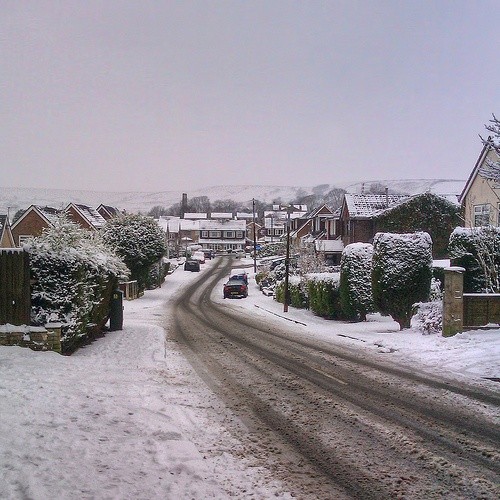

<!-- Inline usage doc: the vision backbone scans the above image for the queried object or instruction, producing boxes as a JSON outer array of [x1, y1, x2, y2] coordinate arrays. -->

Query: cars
[[183, 247, 215, 272], [235, 245, 258, 259], [229, 268, 249, 285], [223, 279, 248, 299]]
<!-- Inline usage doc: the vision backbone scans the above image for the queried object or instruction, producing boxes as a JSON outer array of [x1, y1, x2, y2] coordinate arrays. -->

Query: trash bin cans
[[108, 288, 124, 331]]
